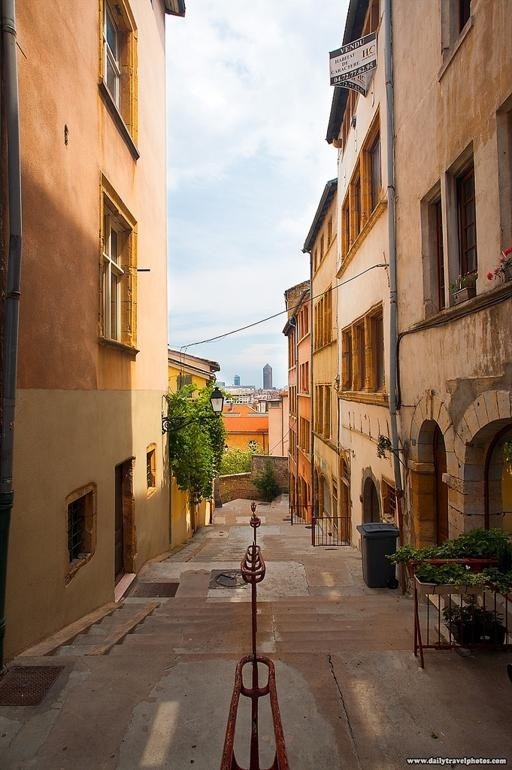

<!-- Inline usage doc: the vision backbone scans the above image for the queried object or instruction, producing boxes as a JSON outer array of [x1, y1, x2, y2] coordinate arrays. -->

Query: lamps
[[160, 386, 223, 435]]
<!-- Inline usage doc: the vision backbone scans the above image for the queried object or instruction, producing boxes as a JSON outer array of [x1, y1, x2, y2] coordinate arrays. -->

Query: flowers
[[487, 247, 511, 281]]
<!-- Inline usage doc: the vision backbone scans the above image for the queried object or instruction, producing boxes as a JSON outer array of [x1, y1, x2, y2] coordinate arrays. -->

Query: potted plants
[[396, 526, 511, 671]]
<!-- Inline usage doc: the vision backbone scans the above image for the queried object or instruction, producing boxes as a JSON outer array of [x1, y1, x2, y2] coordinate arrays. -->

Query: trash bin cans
[[357, 522, 399, 589]]
